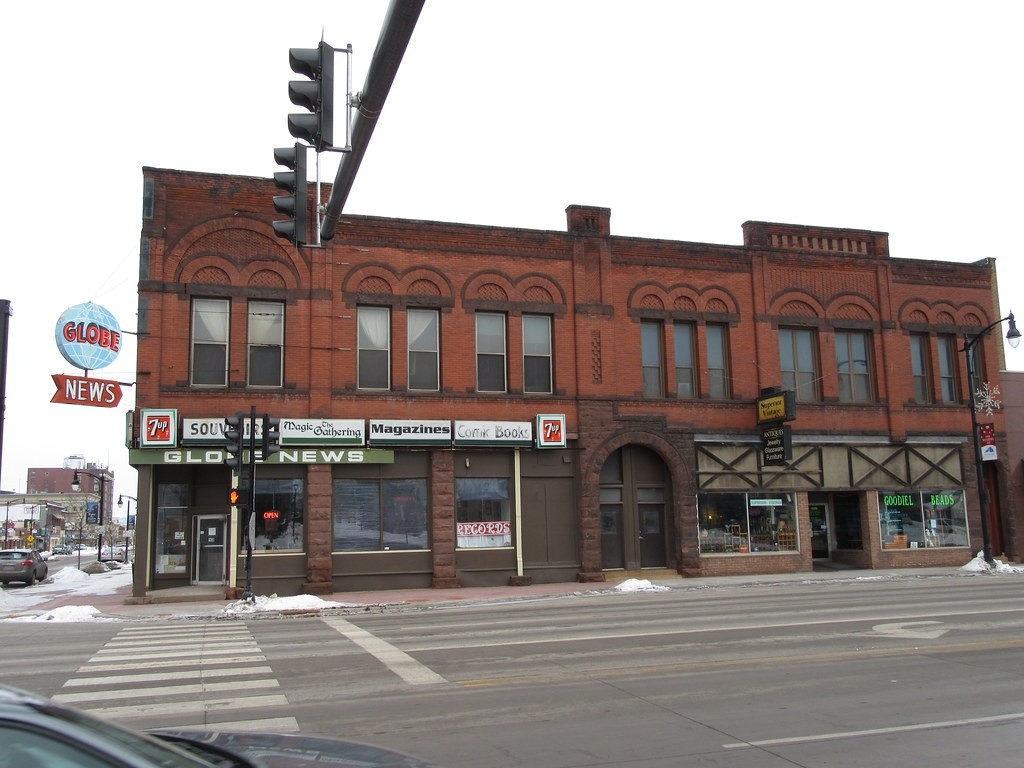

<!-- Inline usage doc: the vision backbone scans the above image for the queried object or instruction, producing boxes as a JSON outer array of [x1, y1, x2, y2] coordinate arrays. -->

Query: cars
[[74, 544, 88, 550], [0, 550, 48, 586], [0, 684, 439, 768], [53, 544, 71, 555], [101, 548, 125, 562]]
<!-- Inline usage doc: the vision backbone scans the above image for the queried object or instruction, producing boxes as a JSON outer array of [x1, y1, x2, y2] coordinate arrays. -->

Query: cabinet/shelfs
[[925, 508, 952, 546], [883, 511, 902, 532], [700, 525, 796, 553]]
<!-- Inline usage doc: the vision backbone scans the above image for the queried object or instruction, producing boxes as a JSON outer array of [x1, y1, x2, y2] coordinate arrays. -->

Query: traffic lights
[[224, 411, 244, 476], [263, 413, 281, 460], [288, 41, 333, 152], [273, 143, 307, 246], [229, 489, 250, 507]]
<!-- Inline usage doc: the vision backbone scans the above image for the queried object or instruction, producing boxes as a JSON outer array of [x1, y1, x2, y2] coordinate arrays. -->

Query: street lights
[[71, 468, 103, 562], [30, 501, 49, 552], [960, 307, 1022, 570], [4, 498, 26, 549], [117, 494, 129, 563]]
